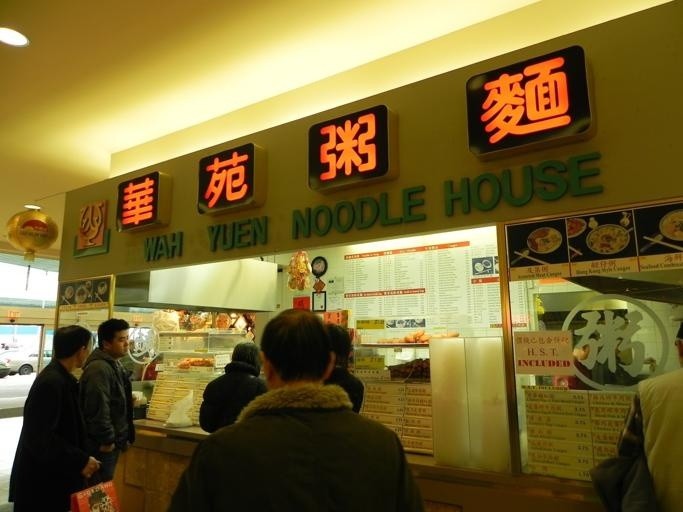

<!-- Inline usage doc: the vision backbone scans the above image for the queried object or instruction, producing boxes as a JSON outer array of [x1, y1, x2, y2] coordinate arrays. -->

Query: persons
[[166, 307, 424, 512], [346, 327, 373, 372], [551, 339, 593, 389], [197, 342, 269, 432], [8, 324, 102, 511], [323, 320, 364, 414], [616, 318, 682, 512], [77, 317, 138, 487]]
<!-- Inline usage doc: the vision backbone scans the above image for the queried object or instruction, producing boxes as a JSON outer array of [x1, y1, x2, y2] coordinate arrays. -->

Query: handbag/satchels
[[588, 458, 656, 512], [71, 480, 120, 512]]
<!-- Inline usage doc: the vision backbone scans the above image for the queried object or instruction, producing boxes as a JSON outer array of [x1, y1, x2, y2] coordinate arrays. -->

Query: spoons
[[509, 250, 530, 267]]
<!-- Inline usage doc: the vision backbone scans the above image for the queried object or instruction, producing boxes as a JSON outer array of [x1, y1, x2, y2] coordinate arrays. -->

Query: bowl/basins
[[526, 208, 683, 255]]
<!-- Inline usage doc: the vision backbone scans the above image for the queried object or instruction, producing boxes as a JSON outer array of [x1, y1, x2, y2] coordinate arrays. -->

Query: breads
[[405, 329, 459, 343]]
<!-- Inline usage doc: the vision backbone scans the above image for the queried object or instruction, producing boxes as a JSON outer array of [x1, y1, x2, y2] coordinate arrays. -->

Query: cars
[[1, 348, 52, 375]]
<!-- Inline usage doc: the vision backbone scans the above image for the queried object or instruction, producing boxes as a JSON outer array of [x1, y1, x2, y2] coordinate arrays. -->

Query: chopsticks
[[514, 251, 551, 264]]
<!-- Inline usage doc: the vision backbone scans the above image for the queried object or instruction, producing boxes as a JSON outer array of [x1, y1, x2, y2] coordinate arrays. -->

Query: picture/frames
[[311, 256, 327, 278], [312, 291, 326, 312]]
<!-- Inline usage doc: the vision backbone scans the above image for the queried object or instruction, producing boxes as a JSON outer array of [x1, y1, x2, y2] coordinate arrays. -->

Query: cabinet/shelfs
[[357, 342, 429, 383]]
